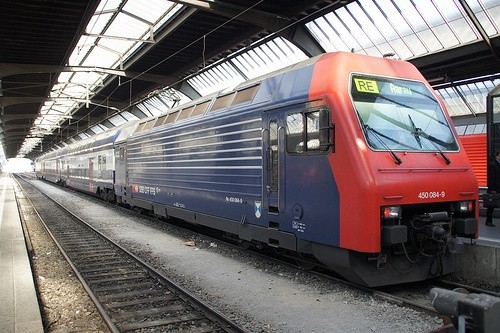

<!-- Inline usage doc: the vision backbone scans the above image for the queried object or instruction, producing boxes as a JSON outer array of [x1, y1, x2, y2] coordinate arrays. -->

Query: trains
[[34, 52, 479, 287]]
